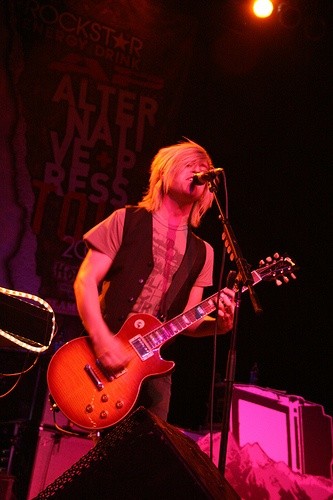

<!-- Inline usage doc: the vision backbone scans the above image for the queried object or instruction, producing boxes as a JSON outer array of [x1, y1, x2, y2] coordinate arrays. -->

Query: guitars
[[45, 252, 298, 430]]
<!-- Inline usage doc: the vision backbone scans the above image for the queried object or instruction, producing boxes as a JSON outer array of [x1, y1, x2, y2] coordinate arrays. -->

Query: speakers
[[32, 406, 242, 500]]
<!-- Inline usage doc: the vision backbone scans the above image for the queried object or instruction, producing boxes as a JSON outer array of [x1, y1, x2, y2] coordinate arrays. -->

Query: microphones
[[193, 168, 222, 185]]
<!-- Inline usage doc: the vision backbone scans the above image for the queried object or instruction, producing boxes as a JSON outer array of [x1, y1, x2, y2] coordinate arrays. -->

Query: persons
[[73, 143, 236, 447]]
